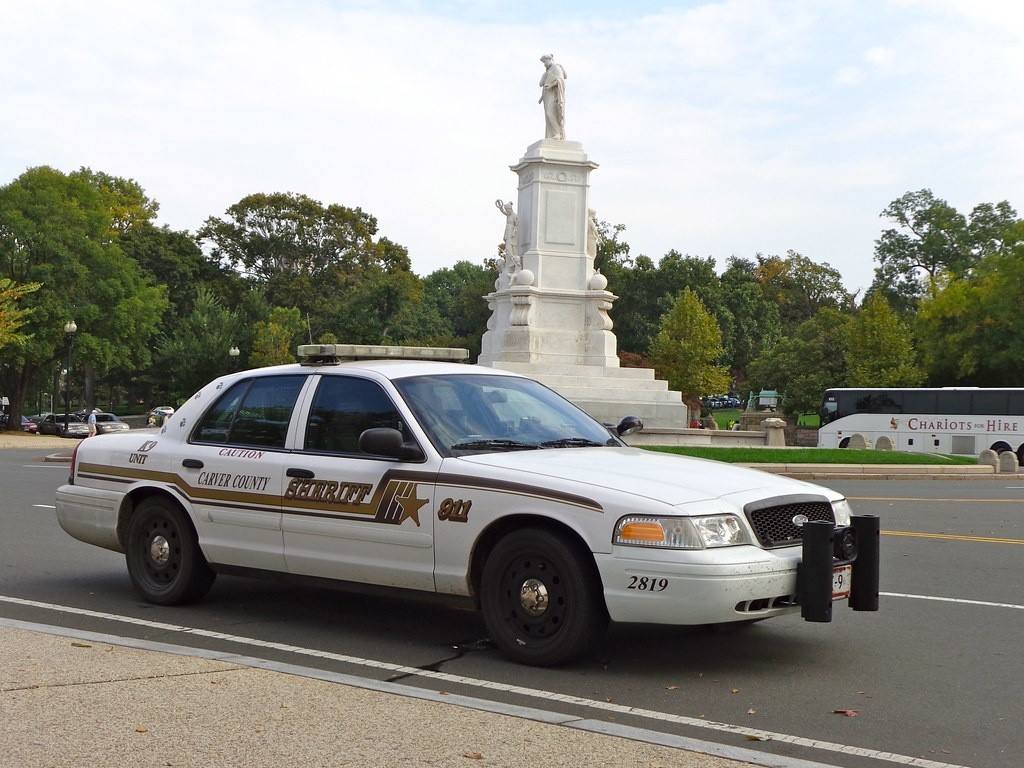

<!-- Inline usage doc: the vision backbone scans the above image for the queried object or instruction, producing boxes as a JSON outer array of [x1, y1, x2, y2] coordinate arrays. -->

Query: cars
[[80, 412, 130, 433], [38, 413, 92, 437], [698, 396, 741, 410], [54, 341, 884, 669], [0, 413, 38, 434], [145, 406, 175, 419]]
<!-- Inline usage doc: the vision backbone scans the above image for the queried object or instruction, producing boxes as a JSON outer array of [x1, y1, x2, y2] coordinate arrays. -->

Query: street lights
[[230, 345, 239, 373], [59, 320, 77, 440]]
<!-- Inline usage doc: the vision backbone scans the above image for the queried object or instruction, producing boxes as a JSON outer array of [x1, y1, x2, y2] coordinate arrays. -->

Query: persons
[[146, 415, 156, 428], [507, 256, 523, 285], [538, 54, 567, 141], [495, 199, 520, 273], [726, 418, 740, 432], [159, 413, 173, 428], [691, 417, 705, 429], [497, 259, 506, 290], [88, 410, 98, 437], [588, 209, 606, 258]]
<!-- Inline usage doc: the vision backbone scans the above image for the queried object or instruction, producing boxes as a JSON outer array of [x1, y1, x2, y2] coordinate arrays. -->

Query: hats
[[150, 412, 157, 416], [92, 410, 97, 412]]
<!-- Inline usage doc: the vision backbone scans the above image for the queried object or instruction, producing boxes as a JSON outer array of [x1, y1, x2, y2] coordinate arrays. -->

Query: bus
[[817, 388, 1024, 466]]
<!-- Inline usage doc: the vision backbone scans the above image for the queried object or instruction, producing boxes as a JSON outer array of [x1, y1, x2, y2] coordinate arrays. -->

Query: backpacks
[[146, 417, 150, 425]]
